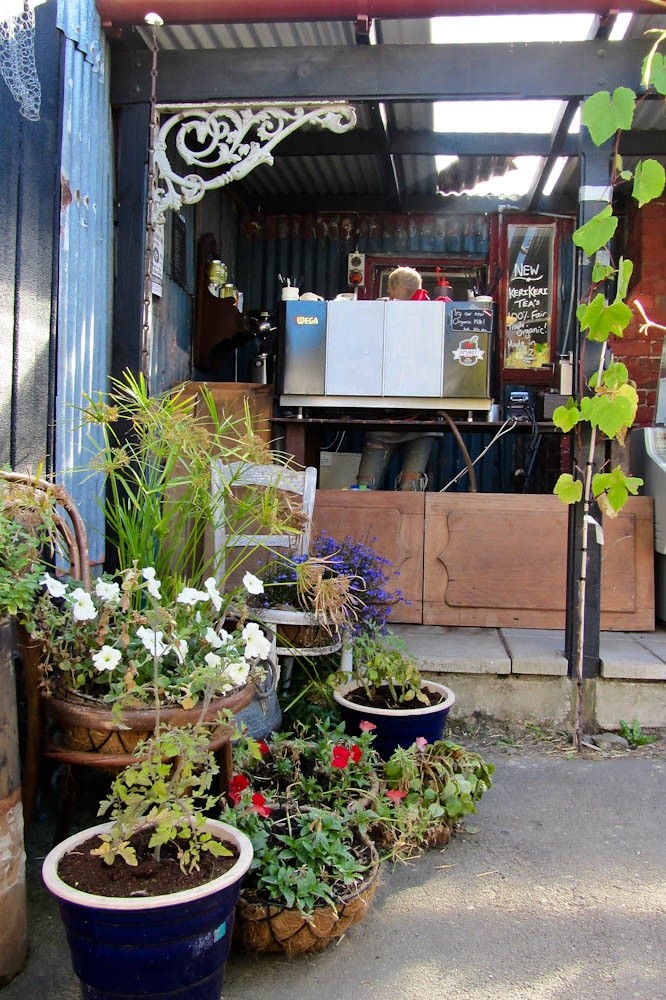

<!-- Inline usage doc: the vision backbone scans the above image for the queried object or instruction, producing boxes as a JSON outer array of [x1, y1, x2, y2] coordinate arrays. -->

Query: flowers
[[32, 566, 272, 727], [229, 723, 412, 823]]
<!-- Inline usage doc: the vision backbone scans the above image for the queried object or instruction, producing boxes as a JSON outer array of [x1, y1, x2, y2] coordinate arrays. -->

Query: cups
[[486, 404, 501, 423], [280, 287, 300, 301], [336, 293, 357, 301], [468, 296, 496, 317]]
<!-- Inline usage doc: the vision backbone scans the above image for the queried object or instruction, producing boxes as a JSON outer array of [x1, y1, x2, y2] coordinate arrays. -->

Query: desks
[[173, 379, 273, 467]]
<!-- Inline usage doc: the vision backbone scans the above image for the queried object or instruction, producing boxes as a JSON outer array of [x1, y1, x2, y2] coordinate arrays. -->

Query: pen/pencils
[[278, 274, 298, 287]]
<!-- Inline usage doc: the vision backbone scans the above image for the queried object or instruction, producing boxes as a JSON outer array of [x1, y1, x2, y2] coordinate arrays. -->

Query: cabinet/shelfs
[[194, 233, 279, 380]]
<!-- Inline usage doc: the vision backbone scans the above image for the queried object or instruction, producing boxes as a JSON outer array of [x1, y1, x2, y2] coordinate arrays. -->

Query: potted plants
[[0, 369, 493, 1000]]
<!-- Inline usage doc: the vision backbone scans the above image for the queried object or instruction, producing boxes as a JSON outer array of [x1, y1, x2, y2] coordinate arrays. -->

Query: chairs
[[204, 459, 355, 690]]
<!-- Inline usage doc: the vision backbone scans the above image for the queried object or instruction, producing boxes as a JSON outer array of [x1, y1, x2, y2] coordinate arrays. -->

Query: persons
[[355, 266, 443, 491]]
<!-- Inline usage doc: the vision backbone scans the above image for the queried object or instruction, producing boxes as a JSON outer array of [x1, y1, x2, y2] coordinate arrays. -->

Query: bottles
[[210, 259, 229, 284], [410, 289, 430, 301], [219, 284, 244, 313], [433, 266, 453, 302]]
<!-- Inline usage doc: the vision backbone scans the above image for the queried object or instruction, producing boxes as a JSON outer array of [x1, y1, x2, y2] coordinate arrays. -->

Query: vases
[[44, 686, 258, 770], [232, 741, 381, 815]]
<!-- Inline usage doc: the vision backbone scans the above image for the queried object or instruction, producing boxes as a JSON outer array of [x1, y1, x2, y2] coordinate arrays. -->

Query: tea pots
[[301, 292, 325, 302]]
